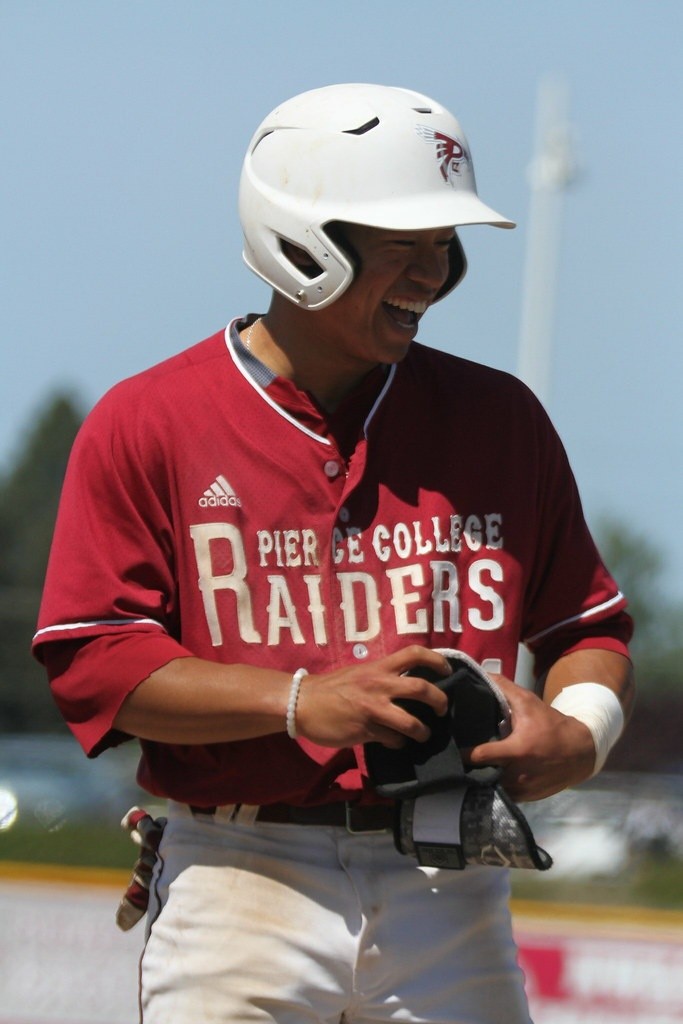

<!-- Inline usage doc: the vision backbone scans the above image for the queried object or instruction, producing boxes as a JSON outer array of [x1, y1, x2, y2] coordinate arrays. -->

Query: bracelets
[[551, 683, 625, 775], [287, 668, 309, 739]]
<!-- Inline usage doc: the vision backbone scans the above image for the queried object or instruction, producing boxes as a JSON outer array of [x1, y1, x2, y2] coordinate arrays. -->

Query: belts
[[188, 801, 394, 836]]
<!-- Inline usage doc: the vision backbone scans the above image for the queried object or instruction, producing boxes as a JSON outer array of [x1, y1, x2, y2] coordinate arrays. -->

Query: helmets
[[237, 81, 516, 310]]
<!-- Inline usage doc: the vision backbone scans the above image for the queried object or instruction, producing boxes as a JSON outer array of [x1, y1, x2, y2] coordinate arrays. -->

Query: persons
[[31, 84, 635, 1024]]
